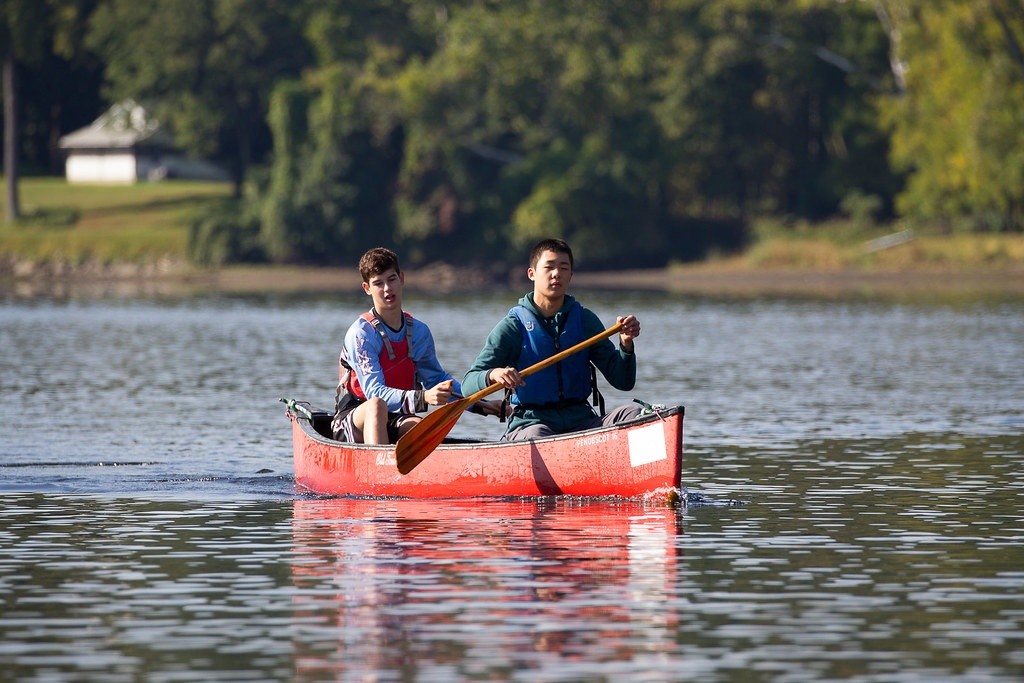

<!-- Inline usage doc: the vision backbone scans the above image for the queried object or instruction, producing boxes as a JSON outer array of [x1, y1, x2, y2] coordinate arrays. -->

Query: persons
[[332, 247, 512, 445], [461, 238, 642, 440]]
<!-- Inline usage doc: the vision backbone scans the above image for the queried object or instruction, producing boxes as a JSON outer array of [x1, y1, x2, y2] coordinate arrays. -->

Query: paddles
[[446, 386, 511, 418], [395, 324, 625, 475]]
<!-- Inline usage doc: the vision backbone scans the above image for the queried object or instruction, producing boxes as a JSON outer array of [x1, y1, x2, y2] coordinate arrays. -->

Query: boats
[[279, 398, 686, 502]]
